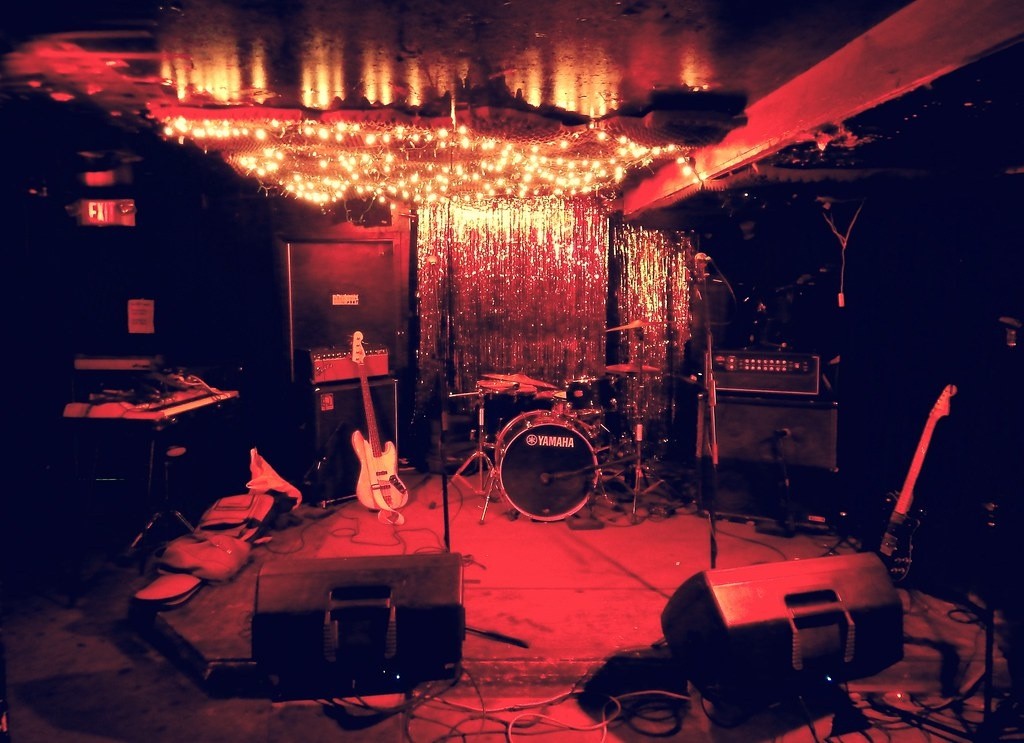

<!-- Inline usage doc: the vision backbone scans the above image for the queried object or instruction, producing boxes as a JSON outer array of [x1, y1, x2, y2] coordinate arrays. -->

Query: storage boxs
[[293, 342, 391, 385]]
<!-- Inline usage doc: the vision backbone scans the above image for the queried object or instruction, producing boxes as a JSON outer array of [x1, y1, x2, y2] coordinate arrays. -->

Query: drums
[[565, 377, 612, 413], [495, 409, 599, 521]]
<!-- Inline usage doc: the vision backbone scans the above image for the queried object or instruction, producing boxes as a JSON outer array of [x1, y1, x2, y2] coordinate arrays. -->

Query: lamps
[[656, 551, 907, 687], [249, 549, 467, 688]]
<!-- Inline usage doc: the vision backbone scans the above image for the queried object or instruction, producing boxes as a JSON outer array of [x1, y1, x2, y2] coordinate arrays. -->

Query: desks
[[60, 391, 243, 562]]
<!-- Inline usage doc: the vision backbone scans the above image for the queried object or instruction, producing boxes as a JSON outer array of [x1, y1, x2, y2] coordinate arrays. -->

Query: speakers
[[250, 553, 467, 699], [699, 396, 851, 535], [660, 551, 903, 708], [297, 378, 398, 507]]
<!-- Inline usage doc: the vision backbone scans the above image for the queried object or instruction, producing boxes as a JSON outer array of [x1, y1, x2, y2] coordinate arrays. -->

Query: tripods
[[575, 327, 698, 525], [428, 393, 503, 509]]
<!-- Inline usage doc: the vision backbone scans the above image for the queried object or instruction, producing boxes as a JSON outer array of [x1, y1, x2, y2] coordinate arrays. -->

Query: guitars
[[350, 331, 409, 511], [866, 384, 958, 583]]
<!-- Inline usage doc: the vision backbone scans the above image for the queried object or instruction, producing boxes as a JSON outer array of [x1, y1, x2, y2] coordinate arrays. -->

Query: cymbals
[[481, 367, 558, 390], [477, 380, 537, 394], [554, 390, 583, 400], [604, 362, 661, 374], [604, 319, 677, 332]]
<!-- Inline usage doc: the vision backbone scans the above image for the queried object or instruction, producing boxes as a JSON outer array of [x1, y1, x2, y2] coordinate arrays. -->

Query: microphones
[[767, 428, 792, 442]]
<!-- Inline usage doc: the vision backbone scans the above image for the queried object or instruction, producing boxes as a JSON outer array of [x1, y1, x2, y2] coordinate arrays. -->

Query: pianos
[[61, 382, 239, 421]]
